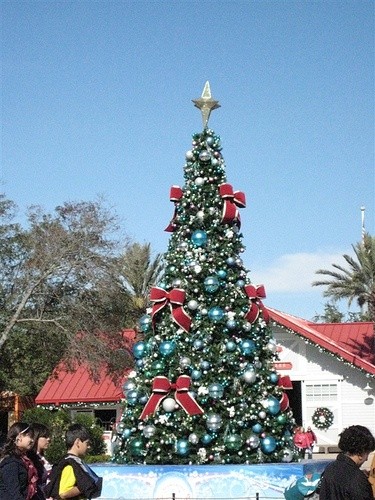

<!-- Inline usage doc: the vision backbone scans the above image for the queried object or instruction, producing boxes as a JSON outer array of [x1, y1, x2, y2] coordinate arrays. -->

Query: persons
[[0, 422, 35, 500], [56, 423, 97, 500], [27, 423, 52, 500], [319, 425, 375, 500], [293, 426, 318, 459]]
